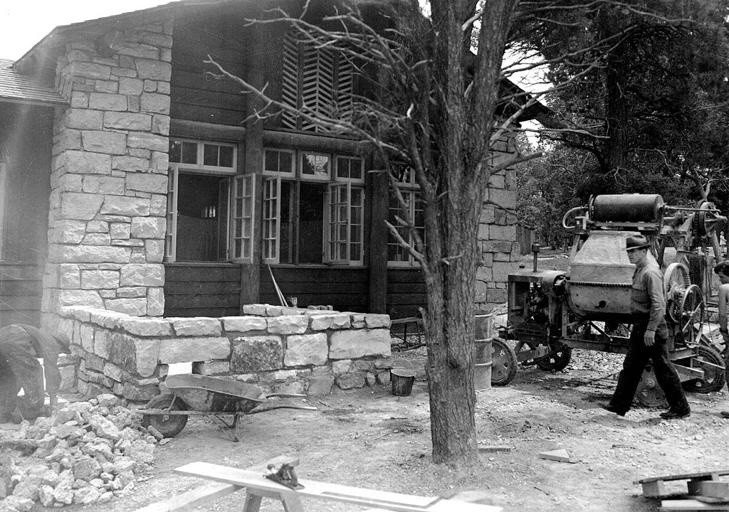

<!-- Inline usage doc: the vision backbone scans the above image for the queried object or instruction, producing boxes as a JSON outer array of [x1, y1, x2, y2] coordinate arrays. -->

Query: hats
[[626, 235, 651, 250], [51, 328, 71, 354]]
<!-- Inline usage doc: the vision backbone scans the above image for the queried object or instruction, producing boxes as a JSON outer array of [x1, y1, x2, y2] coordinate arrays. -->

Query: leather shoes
[[660, 406, 690, 419], [599, 402, 625, 415]]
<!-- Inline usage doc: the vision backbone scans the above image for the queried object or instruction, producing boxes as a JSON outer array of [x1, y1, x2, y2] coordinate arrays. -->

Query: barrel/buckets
[[388, 358, 417, 397], [474, 308, 494, 391]]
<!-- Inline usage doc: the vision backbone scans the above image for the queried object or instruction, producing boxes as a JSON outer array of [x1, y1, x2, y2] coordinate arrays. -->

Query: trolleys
[[134, 373, 317, 444]]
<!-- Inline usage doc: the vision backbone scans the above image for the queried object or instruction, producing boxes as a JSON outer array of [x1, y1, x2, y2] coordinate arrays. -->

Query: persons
[[597, 234, 691, 420], [0, 324, 71, 423], [719, 231, 727, 253], [714, 265, 729, 419]]
